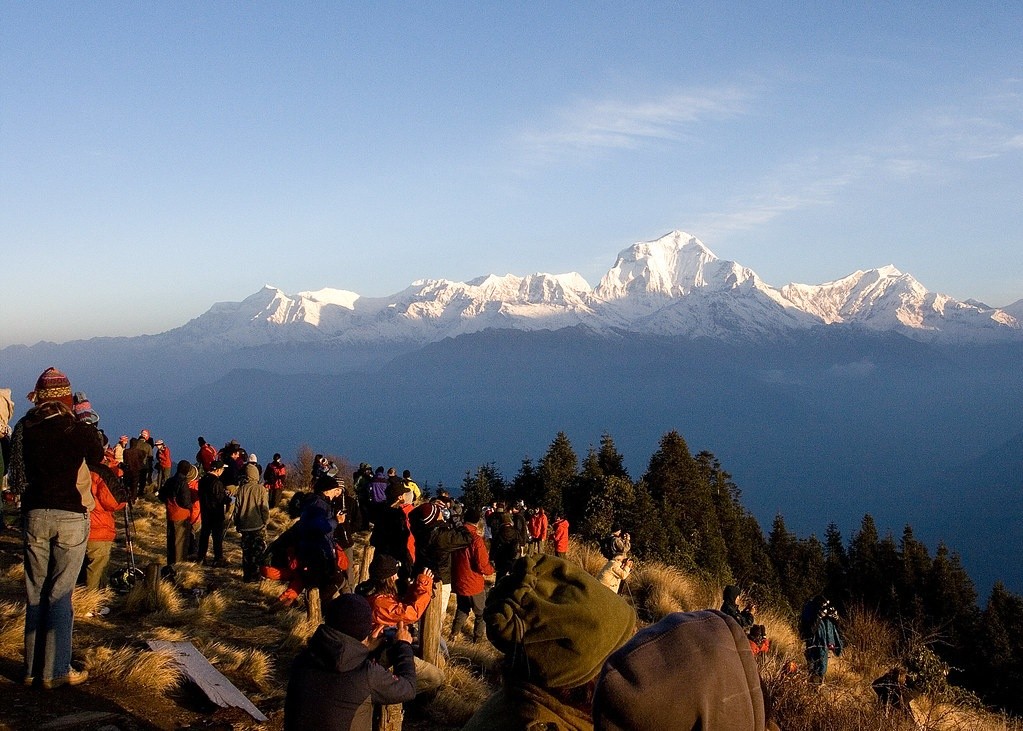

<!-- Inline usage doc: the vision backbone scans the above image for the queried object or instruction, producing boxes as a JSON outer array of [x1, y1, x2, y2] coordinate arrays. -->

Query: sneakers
[[25, 668, 88, 689]]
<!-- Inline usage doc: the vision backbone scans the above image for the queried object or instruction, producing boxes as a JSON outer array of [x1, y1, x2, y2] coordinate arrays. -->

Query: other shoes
[[155, 491, 160, 496], [84, 604, 110, 617]]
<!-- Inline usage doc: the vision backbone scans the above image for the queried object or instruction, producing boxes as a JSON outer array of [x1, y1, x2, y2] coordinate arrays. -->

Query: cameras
[[384, 624, 417, 647], [826, 608, 835, 614]]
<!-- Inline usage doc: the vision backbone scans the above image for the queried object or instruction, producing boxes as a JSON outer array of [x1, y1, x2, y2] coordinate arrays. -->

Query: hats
[[119, 434, 130, 442], [314, 475, 339, 492], [0, 387, 15, 438], [335, 476, 345, 492], [325, 593, 373, 641], [369, 555, 399, 579], [198, 437, 206, 447], [210, 461, 229, 470], [26, 367, 73, 411], [383, 483, 410, 502], [417, 503, 441, 525], [248, 453, 258, 462], [155, 440, 164, 445]]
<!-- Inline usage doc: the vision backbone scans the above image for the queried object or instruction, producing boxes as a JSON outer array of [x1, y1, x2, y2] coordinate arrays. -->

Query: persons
[[283, 555, 443, 731], [798, 592, 843, 689], [871, 667, 929, 724], [288, 454, 570, 647], [86, 408, 287, 594], [9, 368, 104, 689], [462, 552, 776, 731], [598, 524, 633, 594], [0, 389, 19, 537]]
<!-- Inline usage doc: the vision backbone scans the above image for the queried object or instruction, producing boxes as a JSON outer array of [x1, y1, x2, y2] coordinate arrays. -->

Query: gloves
[[72, 391, 93, 423]]
